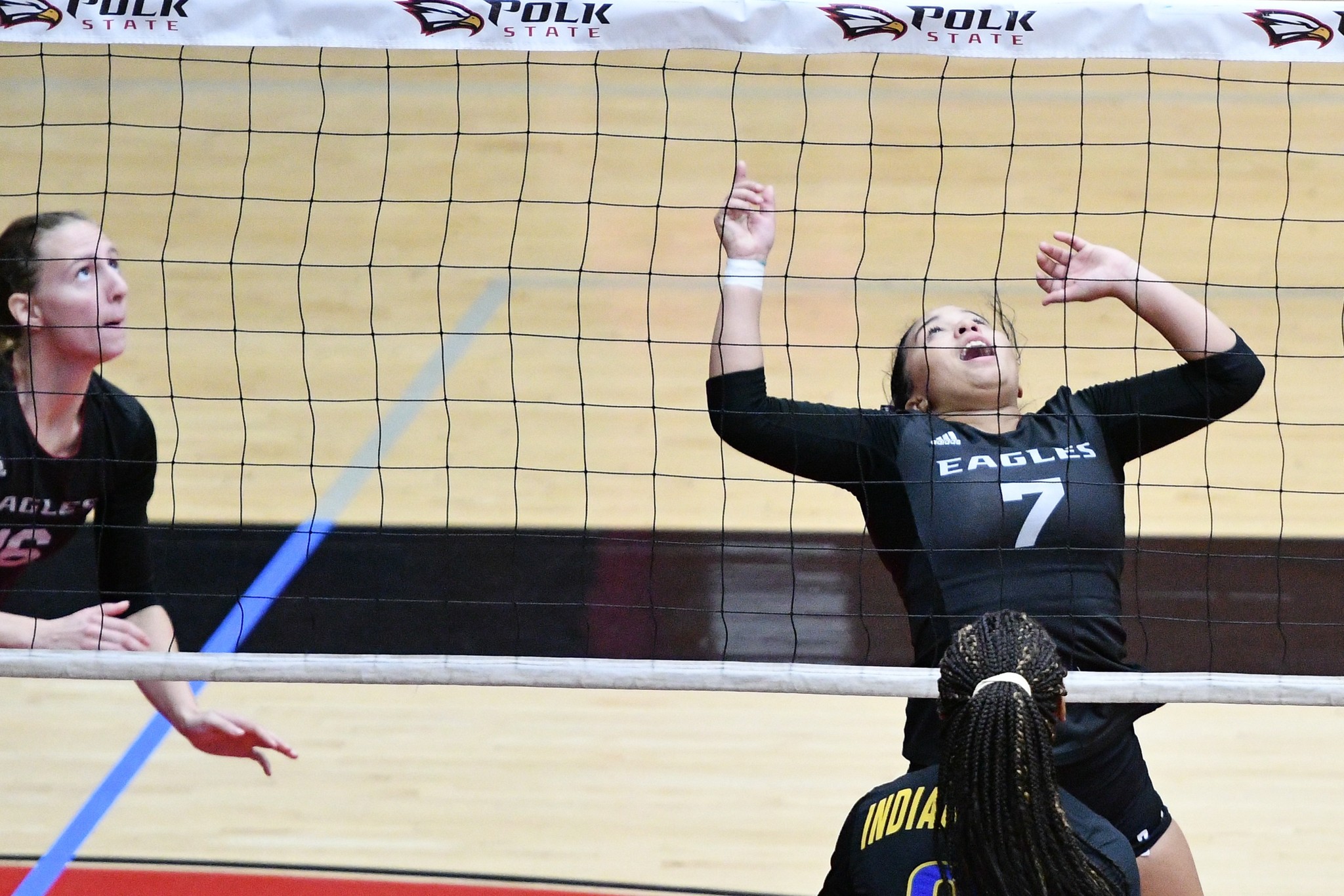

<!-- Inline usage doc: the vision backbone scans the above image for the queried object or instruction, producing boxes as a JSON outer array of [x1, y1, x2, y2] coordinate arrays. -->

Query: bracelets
[[721, 258, 766, 293]]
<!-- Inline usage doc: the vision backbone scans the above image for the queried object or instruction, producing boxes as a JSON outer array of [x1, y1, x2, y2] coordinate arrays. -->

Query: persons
[[705, 157, 1266, 896], [1, 211, 300, 778], [818, 610, 1141, 896]]
